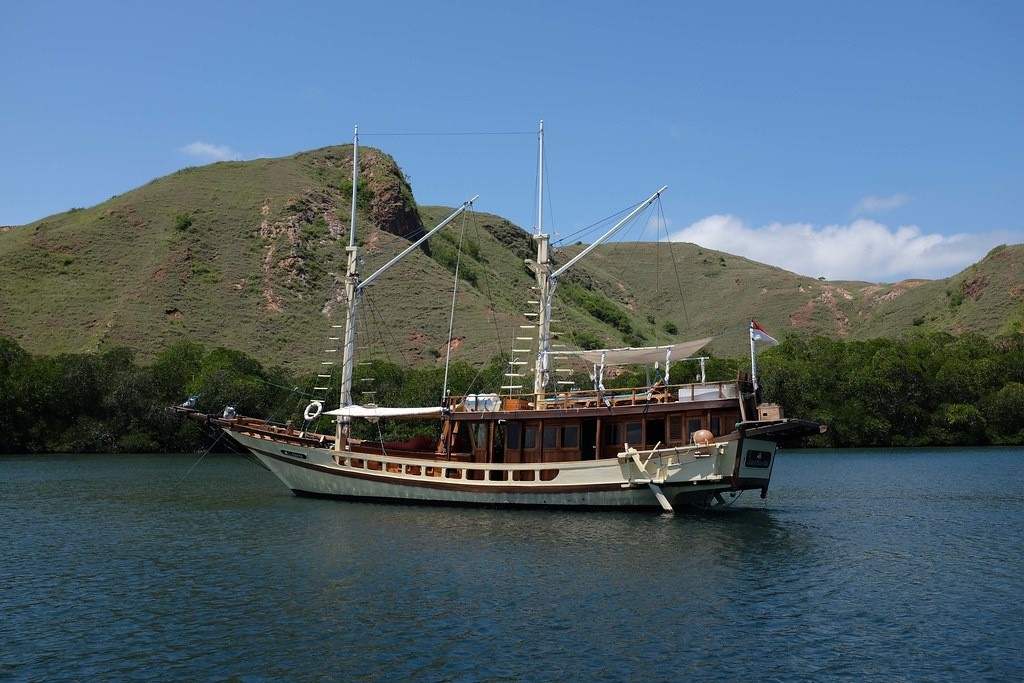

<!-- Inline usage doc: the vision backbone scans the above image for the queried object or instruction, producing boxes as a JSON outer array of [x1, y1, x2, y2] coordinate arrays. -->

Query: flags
[[752, 321, 778, 345]]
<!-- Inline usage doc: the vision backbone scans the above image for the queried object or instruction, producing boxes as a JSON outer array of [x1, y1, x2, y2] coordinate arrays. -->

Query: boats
[[164, 119, 828, 514]]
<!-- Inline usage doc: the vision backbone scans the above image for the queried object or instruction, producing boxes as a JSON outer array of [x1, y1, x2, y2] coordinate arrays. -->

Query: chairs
[[538, 380, 671, 410]]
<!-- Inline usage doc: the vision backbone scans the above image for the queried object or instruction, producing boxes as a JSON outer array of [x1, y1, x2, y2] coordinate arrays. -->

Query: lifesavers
[[304, 401, 323, 422]]
